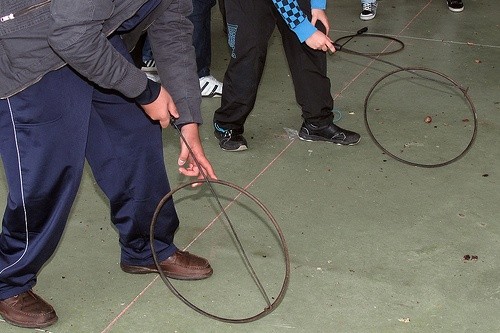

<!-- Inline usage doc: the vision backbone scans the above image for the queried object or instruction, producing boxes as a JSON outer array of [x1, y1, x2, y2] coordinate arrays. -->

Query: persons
[[213, 0, 361, 151], [0, 0, 218, 329], [141, 0, 224, 97], [360, 0, 464, 20]]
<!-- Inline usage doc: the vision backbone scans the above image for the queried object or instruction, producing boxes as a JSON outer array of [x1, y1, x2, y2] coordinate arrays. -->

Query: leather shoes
[[0, 290, 58, 327], [120, 249, 213, 280]]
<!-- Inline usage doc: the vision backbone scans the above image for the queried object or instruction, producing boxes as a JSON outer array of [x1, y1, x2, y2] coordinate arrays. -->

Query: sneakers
[[140, 59, 157, 72], [200, 75, 223, 97], [447, 0, 465, 12], [214, 122, 249, 151], [298, 120, 361, 145], [360, 2, 378, 20]]
